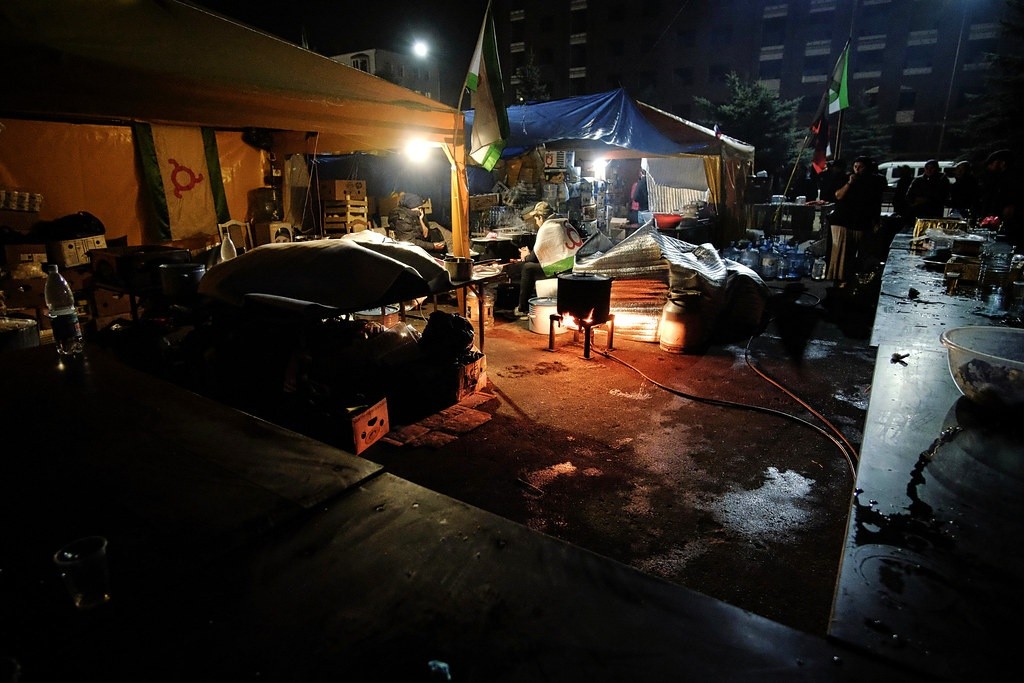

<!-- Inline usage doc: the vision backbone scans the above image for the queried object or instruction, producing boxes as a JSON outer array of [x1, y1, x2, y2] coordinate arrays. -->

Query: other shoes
[[504, 310, 529, 321]]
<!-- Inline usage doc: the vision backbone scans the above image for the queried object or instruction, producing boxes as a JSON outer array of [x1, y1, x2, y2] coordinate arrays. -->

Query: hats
[[529, 202, 553, 216], [985, 150, 1016, 166], [398, 193, 424, 209]]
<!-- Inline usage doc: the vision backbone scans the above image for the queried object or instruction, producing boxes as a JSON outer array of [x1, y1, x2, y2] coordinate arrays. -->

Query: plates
[[446, 251, 480, 258]]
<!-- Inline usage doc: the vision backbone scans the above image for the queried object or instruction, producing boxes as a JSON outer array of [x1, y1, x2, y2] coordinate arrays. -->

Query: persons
[[511, 201, 583, 317], [891, 149, 1020, 228], [825, 156, 886, 231], [631, 167, 648, 222], [389, 192, 448, 259]]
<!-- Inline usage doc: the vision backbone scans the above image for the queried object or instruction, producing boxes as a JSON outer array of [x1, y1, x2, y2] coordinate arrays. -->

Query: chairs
[[217, 220, 254, 250], [344, 219, 369, 233]]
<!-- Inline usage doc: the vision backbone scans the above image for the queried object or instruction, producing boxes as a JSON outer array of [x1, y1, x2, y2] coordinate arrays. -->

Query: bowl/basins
[[443, 257, 474, 282], [641, 212, 653, 223], [652, 212, 682, 228], [925, 260, 946, 272]]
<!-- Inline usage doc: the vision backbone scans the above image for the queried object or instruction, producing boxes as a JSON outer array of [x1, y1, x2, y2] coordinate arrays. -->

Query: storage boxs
[[315, 395, 390, 456], [90, 309, 144, 331], [0, 209, 39, 230], [566, 177, 630, 234], [315, 180, 366, 201], [49, 235, 108, 269], [3, 245, 47, 265], [87, 288, 141, 317], [441, 351, 486, 405], [324, 195, 368, 239]]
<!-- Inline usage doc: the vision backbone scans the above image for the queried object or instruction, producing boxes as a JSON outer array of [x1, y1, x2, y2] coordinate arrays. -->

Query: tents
[[0, 0, 472, 260], [545, 100, 756, 206]]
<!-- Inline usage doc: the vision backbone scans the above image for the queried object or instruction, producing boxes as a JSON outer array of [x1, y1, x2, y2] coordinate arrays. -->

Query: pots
[[527, 296, 568, 334], [158, 263, 206, 295], [493, 282, 519, 308], [554, 270, 616, 321]]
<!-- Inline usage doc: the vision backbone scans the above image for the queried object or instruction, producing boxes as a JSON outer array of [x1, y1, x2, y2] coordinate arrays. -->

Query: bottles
[[804, 251, 812, 275], [479, 206, 510, 226], [659, 289, 704, 354], [77, 300, 88, 313], [44, 265, 84, 354], [723, 237, 804, 279], [367, 219, 372, 229], [473, 192, 490, 207], [220, 232, 236, 262], [812, 257, 826, 279]]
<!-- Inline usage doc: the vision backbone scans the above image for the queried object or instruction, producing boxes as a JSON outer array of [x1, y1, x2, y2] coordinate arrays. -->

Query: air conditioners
[[544, 150, 575, 169]]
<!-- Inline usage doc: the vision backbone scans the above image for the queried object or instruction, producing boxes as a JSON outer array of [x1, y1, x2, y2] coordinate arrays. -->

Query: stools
[[255, 221, 294, 246]]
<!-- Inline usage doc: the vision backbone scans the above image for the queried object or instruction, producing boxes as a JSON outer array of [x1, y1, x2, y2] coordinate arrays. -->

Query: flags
[[464, 4, 511, 172], [813, 115, 833, 172], [825, 46, 850, 114]]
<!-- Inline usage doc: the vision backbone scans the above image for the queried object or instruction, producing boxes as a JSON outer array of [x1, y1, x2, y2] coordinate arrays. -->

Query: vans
[[877, 161, 956, 190]]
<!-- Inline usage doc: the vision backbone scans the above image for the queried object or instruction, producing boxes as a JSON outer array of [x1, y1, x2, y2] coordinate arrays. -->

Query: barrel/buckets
[[354, 305, 400, 329], [466, 291, 494, 335], [543, 183, 569, 198]]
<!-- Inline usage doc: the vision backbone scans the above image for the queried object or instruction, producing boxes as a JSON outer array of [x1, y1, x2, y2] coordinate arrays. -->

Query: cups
[[380, 215, 389, 227], [946, 271, 959, 294], [986, 284, 1003, 314]]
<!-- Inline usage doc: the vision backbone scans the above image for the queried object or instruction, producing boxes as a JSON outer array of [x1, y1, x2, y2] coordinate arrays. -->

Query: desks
[[321, 265, 507, 354], [619, 223, 703, 241], [752, 203, 835, 243]]
